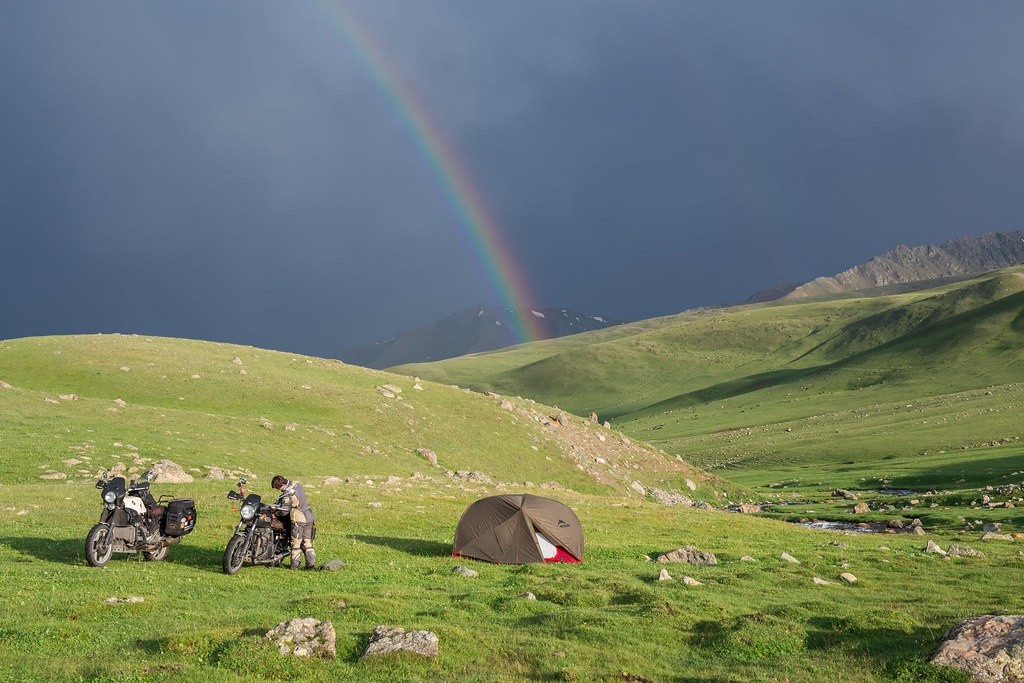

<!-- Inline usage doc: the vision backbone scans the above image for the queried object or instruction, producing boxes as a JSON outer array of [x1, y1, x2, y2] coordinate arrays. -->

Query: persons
[[271, 476, 319, 573]]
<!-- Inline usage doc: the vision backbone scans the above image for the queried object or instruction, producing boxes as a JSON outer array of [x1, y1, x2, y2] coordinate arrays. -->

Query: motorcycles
[[84, 470, 184, 568], [223, 478, 316, 574]]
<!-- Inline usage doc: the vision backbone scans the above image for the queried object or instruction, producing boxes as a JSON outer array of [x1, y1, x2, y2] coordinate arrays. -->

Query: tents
[[453, 492, 586, 566]]
